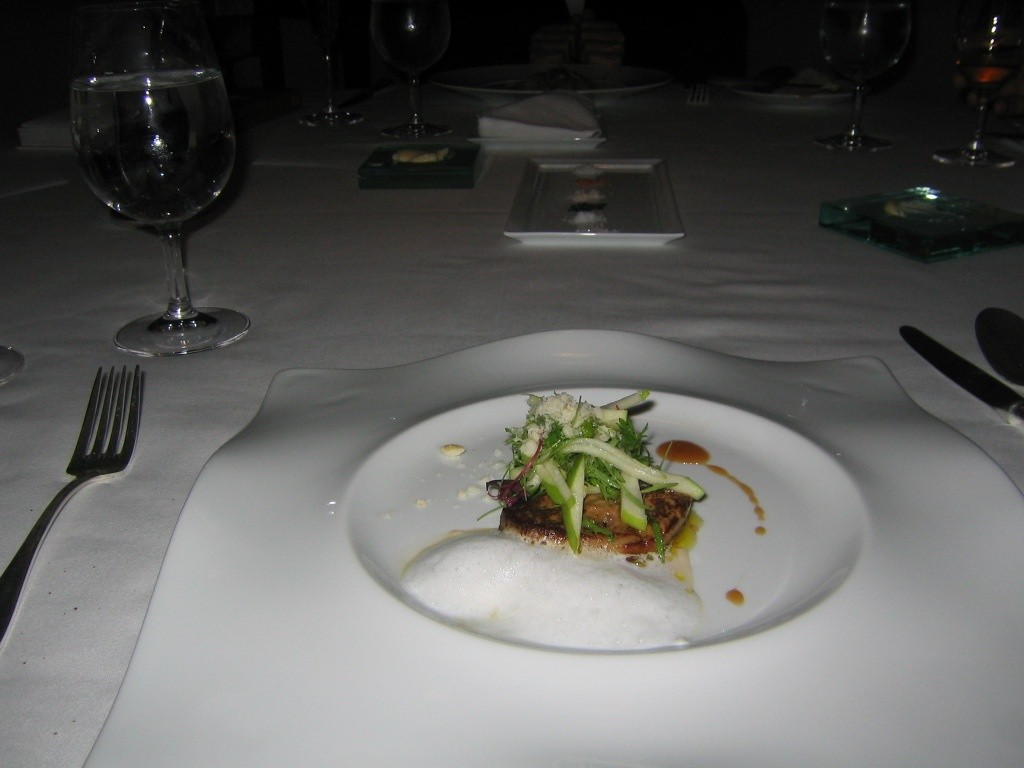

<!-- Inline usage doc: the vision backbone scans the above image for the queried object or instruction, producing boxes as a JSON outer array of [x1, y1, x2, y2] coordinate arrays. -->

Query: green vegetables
[[478, 398, 681, 564]]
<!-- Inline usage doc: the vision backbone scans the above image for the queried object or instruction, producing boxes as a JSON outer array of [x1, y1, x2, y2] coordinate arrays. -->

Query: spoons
[[973, 307, 1024, 385]]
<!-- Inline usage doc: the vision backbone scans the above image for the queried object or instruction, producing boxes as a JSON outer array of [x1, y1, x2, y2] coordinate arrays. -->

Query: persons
[[333, 0, 747, 104], [945, 26, 1024, 119]]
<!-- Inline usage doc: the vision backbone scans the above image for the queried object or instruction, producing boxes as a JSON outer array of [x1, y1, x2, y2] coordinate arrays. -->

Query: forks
[[0, 363, 147, 642], [685, 79, 718, 107]]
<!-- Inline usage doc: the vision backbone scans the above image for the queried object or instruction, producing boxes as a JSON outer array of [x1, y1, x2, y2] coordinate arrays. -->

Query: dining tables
[[0, 80, 1024, 768]]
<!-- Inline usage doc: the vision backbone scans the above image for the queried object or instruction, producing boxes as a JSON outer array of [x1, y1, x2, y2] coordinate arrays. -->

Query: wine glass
[[377, 4, 455, 142], [295, 4, 365, 131], [933, 3, 1024, 169], [71, 68, 252, 356], [811, 2, 912, 158]]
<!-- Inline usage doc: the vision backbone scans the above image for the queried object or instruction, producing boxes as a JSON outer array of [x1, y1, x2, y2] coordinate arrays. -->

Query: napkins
[[474, 94, 603, 140], [774, 66, 852, 93]]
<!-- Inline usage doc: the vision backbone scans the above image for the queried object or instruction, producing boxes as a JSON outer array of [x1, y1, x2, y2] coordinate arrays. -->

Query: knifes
[[898, 322, 1023, 438]]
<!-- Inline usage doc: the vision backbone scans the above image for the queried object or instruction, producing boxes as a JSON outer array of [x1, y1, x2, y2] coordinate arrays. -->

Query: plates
[[85, 329, 1024, 768], [503, 154, 688, 250], [732, 67, 862, 109], [437, 66, 672, 109]]
